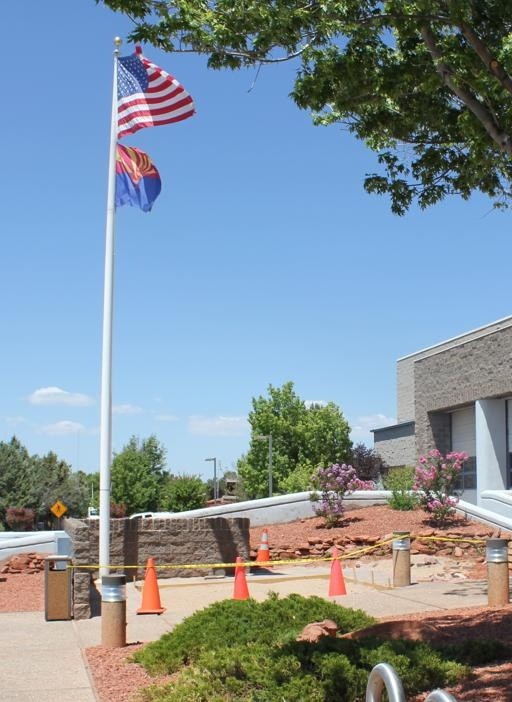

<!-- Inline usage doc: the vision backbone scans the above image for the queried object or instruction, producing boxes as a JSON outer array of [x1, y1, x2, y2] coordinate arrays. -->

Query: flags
[[116, 46, 197, 139], [113, 142, 162, 213]]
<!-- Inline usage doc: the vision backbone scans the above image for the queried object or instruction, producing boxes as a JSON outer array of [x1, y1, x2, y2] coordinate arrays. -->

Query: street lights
[[257, 435, 272, 497], [205, 458, 216, 498]]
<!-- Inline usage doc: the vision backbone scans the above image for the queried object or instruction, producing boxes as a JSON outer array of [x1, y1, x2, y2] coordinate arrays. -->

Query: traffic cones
[[137, 558, 166, 613], [256, 528, 272, 566], [328, 550, 349, 596], [233, 557, 249, 600]]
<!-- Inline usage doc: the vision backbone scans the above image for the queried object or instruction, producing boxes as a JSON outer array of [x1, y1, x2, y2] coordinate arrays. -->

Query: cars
[[87, 509, 99, 519], [129, 513, 153, 519]]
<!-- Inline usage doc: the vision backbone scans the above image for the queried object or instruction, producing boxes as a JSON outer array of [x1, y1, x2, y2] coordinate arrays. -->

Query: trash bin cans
[[44, 555, 72, 621]]
